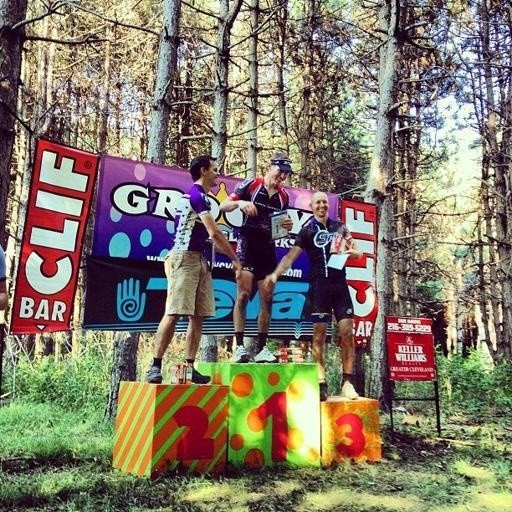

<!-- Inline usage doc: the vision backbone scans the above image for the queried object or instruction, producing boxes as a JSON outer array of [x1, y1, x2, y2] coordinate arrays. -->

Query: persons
[[143, 154, 246, 384], [261, 191, 362, 401], [219, 153, 294, 363], [0, 247, 8, 312]]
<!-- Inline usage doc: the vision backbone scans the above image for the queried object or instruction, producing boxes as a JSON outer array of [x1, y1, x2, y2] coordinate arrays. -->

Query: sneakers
[[253, 344, 278, 363], [341, 381, 359, 400], [234, 345, 250, 362], [146, 366, 162, 383], [192, 367, 210, 384], [320, 379, 329, 400]]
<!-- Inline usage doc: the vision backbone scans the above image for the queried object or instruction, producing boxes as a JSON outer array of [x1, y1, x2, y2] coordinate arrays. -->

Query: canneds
[[278, 348, 288, 364], [171, 363, 193, 385]]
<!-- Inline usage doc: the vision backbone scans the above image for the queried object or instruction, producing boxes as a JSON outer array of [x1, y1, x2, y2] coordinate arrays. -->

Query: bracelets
[[270, 271, 277, 284]]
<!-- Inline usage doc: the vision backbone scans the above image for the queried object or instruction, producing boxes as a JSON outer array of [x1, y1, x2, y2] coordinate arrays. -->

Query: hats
[[271, 158, 295, 175]]
